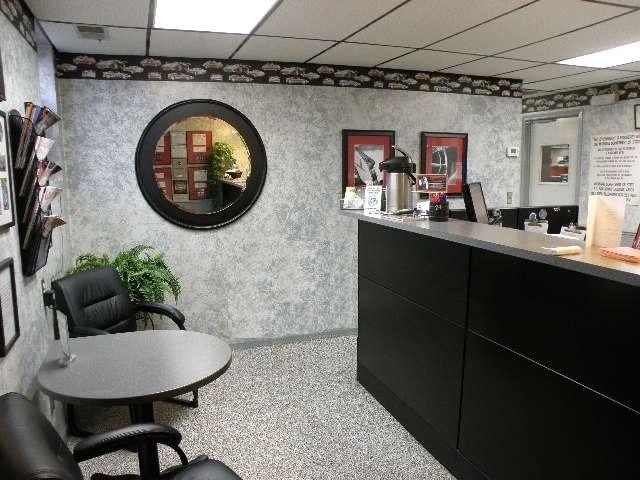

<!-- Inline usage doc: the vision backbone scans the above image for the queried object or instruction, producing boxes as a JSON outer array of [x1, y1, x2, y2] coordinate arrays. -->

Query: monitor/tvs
[[462, 182, 489, 224]]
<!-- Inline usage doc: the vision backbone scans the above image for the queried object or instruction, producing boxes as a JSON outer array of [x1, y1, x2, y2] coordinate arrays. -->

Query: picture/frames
[[0, 257, 20, 357], [342, 129, 395, 200], [0, 110, 15, 231], [420, 131, 468, 200]]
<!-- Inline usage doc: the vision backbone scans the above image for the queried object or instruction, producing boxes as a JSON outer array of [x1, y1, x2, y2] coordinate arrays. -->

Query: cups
[[429, 201, 450, 222]]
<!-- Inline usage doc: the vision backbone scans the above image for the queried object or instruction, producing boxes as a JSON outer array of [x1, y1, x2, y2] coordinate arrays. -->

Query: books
[[14, 100, 69, 273]]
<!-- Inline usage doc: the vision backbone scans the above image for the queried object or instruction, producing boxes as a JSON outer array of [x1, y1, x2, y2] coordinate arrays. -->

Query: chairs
[[0, 391, 243, 480], [51, 265, 199, 453]]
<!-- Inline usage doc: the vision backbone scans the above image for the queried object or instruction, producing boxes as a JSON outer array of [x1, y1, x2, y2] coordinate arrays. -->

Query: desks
[[36, 329, 232, 476]]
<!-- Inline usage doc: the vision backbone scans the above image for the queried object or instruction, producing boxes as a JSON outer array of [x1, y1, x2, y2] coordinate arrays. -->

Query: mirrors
[[135, 99, 268, 230]]
